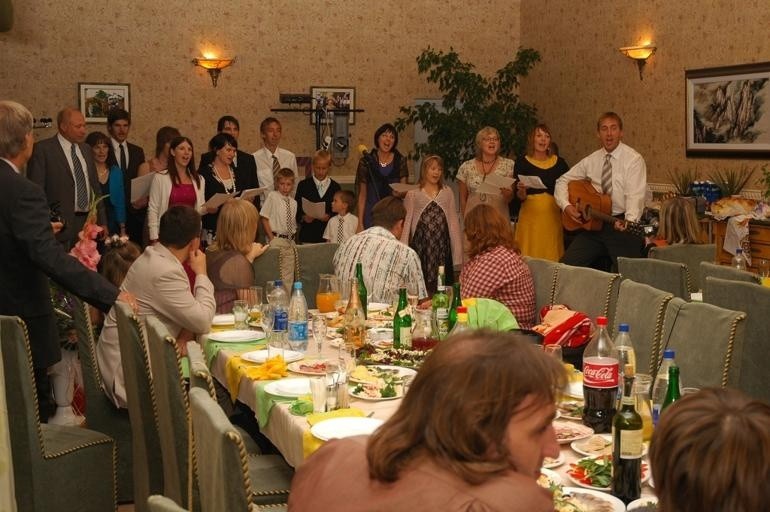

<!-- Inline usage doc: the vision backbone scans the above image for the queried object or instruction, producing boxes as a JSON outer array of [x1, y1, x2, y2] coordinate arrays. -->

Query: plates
[[366, 301, 390, 311], [348, 384, 404, 400], [556, 399, 585, 420], [245, 317, 262, 327], [211, 314, 235, 325], [262, 376, 311, 396], [550, 407, 560, 421], [536, 467, 562, 489], [552, 488, 626, 512], [540, 451, 566, 468], [561, 381, 585, 399], [374, 339, 394, 348], [625, 494, 662, 512], [648, 476, 656, 490], [326, 328, 341, 338], [310, 417, 386, 442], [571, 432, 648, 457], [327, 339, 343, 348], [240, 348, 305, 364], [205, 330, 266, 343], [288, 357, 341, 375], [548, 421, 595, 442], [565, 452, 652, 490], [350, 365, 420, 385]]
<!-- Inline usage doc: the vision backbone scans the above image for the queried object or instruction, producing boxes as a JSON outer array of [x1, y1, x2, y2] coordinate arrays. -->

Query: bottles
[[315, 273, 340, 313], [653, 348, 679, 426], [730, 248, 745, 270], [449, 305, 472, 341], [612, 363, 642, 501], [432, 265, 449, 342], [581, 315, 620, 432], [352, 262, 367, 317], [269, 280, 289, 332], [286, 281, 309, 350], [447, 282, 464, 333], [326, 382, 351, 413], [199, 229, 217, 253], [392, 287, 413, 351], [342, 277, 367, 349], [633, 373, 655, 441], [614, 322, 638, 375], [661, 365, 681, 408]]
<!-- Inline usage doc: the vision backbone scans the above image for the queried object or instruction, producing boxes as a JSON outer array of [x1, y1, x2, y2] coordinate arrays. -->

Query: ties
[[119, 144, 127, 172], [601, 154, 614, 197], [317, 183, 323, 199], [70, 143, 90, 210], [270, 155, 281, 190], [337, 218, 344, 245], [284, 198, 292, 240]]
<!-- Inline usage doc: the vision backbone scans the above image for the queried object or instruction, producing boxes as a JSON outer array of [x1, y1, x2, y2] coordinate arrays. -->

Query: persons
[[552, 107, 649, 273], [24, 104, 112, 351], [283, 328, 567, 512], [83, 92, 707, 337], [93, 203, 272, 458], [453, 122, 517, 227], [510, 121, 570, 264], [0, 99, 123, 425], [626, 384, 770, 511]]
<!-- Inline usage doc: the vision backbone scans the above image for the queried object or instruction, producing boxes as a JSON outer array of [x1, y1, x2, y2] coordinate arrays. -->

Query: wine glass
[[311, 314, 327, 353], [259, 304, 276, 333], [338, 342, 356, 382]]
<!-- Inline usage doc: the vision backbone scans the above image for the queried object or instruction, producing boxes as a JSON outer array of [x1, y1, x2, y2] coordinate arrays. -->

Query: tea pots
[[411, 307, 440, 353]]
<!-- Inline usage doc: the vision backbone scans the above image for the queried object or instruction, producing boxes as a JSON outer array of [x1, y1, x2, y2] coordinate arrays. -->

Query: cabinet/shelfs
[[714, 217, 770, 279]]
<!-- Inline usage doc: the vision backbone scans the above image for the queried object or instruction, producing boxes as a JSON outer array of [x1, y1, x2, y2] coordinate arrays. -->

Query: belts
[[75, 212, 89, 215], [271, 231, 297, 239]]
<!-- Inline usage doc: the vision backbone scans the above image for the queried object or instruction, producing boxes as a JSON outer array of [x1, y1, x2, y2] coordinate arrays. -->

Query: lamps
[[618, 38, 659, 81], [192, 45, 236, 87]]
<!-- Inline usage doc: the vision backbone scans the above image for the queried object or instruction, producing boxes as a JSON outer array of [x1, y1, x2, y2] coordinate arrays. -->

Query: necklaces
[[478, 157, 500, 183]]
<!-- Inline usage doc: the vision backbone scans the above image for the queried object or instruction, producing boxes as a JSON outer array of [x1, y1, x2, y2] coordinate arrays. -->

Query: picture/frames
[[310, 85, 356, 125], [77, 81, 132, 125], [683, 62, 770, 159]]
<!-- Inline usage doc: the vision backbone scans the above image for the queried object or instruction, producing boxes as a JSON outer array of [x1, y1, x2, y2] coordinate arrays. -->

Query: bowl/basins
[[367, 328, 394, 342]]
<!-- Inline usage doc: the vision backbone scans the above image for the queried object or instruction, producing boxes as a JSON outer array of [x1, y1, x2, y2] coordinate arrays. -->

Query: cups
[[232, 299, 249, 330], [265, 330, 285, 373], [535, 344, 544, 351], [759, 259, 770, 288], [248, 286, 263, 318], [545, 344, 563, 361], [310, 377, 327, 413], [266, 281, 276, 304]]
